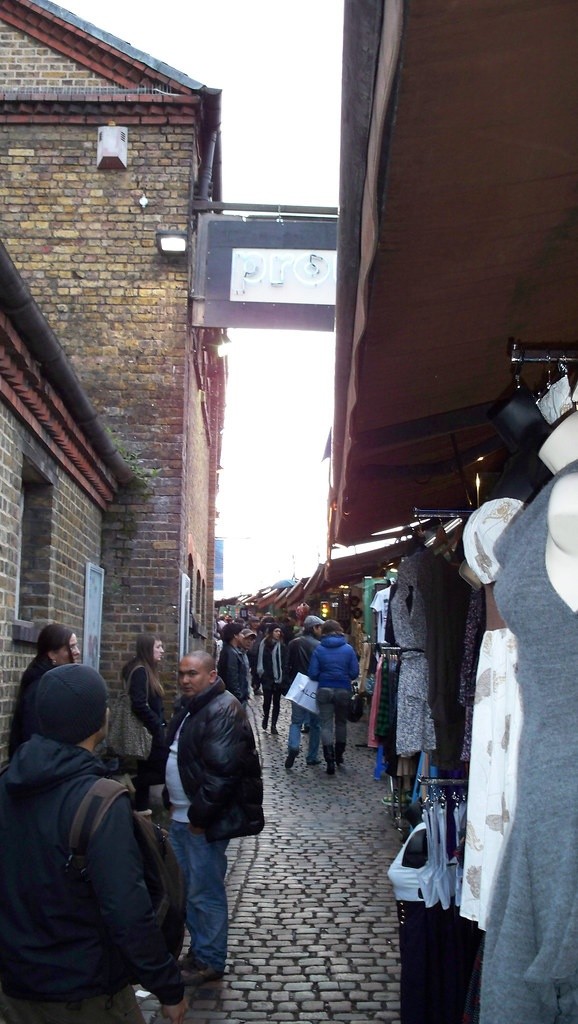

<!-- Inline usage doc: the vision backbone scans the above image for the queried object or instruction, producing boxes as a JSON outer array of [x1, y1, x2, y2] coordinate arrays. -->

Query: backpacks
[[65, 777, 188, 984]]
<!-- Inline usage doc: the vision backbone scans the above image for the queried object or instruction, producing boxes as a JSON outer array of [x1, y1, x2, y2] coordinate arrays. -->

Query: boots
[[271, 724, 278, 734], [336, 742, 346, 766], [324, 744, 335, 774], [262, 716, 268, 730]]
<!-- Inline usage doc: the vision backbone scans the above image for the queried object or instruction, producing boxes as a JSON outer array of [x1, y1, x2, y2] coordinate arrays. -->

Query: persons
[[284, 614, 325, 770], [478, 404, 578, 1024], [216, 623, 257, 710], [166, 650, 265, 986], [107, 631, 169, 811], [216, 615, 291, 694], [7, 622, 80, 765], [258, 624, 289, 736], [308, 620, 360, 774], [1, 664, 188, 1024]]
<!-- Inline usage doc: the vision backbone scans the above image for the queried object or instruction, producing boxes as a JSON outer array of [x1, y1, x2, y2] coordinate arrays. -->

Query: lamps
[[155, 225, 190, 255]]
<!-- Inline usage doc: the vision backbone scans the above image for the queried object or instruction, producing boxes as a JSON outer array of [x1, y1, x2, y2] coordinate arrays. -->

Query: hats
[[268, 624, 282, 633], [305, 616, 325, 629], [252, 616, 260, 622], [35, 664, 108, 746], [242, 629, 257, 640]]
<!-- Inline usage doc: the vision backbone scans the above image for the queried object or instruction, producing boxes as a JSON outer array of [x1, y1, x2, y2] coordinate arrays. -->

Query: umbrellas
[[271, 579, 296, 589]]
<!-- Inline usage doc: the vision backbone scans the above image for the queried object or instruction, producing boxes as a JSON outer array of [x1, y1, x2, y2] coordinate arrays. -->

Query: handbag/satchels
[[104, 665, 151, 761], [348, 689, 364, 723], [285, 672, 320, 716]]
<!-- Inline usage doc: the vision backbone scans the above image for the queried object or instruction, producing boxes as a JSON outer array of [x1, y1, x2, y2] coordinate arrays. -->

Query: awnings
[[332, 0, 578, 545], [245, 537, 426, 607]]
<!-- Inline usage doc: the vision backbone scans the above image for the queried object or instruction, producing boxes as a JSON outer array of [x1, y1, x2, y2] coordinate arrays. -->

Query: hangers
[[376, 344, 574, 846]]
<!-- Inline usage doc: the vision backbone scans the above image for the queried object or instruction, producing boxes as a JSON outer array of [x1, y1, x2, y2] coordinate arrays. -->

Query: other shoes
[[285, 750, 299, 769], [302, 725, 310, 733], [254, 690, 263, 695], [177, 954, 222, 985]]
